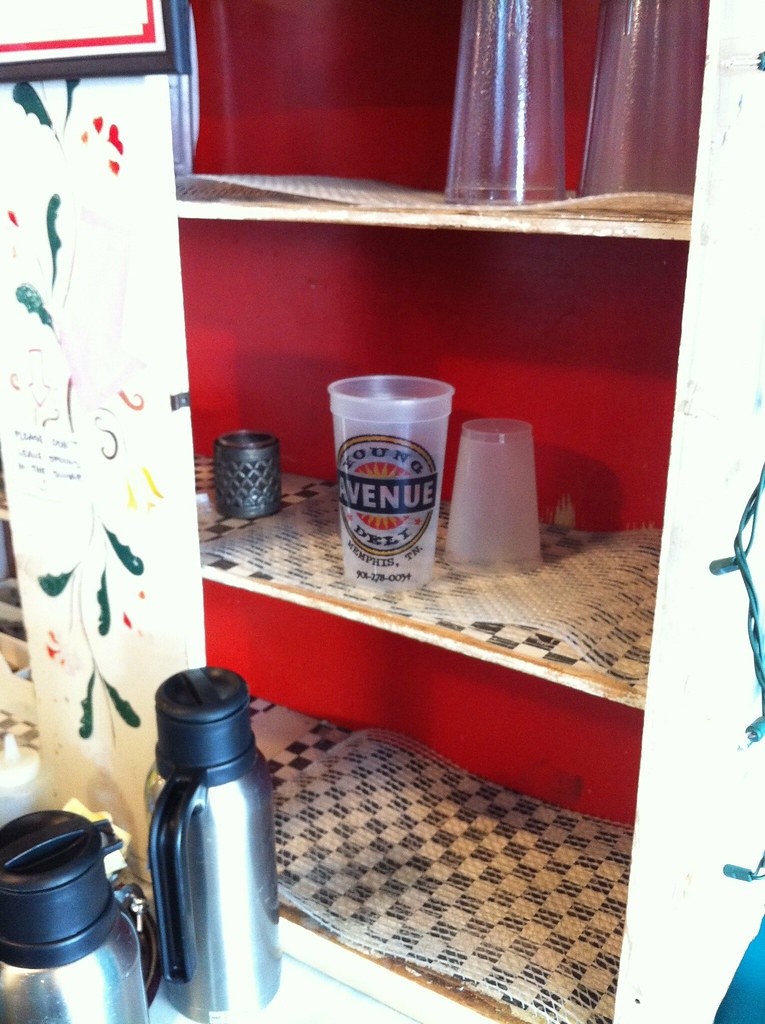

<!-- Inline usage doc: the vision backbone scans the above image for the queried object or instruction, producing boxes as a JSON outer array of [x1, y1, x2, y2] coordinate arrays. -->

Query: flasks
[[0, 666, 282, 1024]]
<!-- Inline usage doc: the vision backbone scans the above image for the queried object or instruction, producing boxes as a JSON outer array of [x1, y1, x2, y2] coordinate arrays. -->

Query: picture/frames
[[0, 0, 190, 82]]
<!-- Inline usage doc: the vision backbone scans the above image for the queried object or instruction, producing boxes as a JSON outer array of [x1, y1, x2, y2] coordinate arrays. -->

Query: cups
[[444, 0, 711, 206], [213, 430, 280, 519], [326, 375, 541, 597]]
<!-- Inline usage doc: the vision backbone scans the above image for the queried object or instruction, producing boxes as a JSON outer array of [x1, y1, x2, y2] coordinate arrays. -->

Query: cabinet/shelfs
[[165, 0, 716, 1024]]
